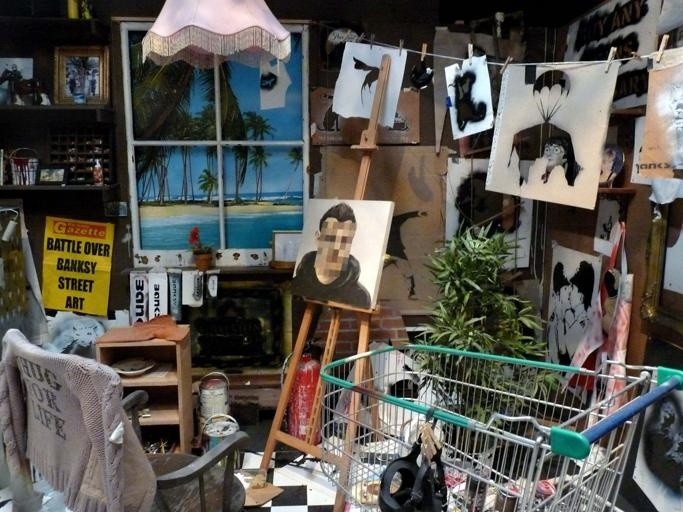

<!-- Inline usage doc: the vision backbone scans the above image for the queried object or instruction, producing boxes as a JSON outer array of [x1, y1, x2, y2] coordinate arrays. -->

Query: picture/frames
[[637, 184, 683, 338], [52, 43, 112, 108], [0, 54, 38, 102]]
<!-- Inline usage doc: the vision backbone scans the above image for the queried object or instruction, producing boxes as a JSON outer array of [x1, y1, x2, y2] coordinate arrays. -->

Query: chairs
[[1, 321, 256, 512]]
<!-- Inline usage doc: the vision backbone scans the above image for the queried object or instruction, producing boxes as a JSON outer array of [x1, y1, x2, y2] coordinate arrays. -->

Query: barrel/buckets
[[199, 371, 232, 419], [199, 414, 241, 470], [8, 148, 40, 186]]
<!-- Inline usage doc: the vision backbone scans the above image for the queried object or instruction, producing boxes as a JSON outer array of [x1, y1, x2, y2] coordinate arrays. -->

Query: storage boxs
[[309, 82, 424, 148]]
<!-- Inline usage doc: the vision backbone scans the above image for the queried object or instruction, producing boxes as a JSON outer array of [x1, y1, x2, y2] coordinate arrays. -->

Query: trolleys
[[312, 340, 682, 512]]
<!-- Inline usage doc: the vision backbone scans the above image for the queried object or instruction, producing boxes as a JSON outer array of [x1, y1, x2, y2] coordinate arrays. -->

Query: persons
[[547, 261, 572, 366], [66, 68, 98, 96], [8, 64, 23, 103], [563, 260, 595, 366], [543, 137, 569, 184], [293, 203, 371, 310]]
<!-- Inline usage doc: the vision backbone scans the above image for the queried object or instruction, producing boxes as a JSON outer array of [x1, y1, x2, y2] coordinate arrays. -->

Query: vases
[[192, 253, 213, 272]]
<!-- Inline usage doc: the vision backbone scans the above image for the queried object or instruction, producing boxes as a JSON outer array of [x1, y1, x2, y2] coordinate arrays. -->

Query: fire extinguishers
[[192, 370, 230, 449], [280, 337, 322, 446]]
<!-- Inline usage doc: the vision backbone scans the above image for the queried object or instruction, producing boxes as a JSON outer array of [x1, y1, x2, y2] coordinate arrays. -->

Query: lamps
[[139, 0, 297, 72]]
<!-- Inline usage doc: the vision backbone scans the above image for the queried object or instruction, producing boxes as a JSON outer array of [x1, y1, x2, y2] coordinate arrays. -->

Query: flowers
[[183, 224, 215, 252]]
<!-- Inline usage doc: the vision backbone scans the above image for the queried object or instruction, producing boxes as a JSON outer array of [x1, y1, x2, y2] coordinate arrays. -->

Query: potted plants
[[408, 197, 562, 483]]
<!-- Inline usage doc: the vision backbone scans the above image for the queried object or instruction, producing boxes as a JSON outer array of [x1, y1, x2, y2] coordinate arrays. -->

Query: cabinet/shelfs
[[93, 320, 193, 457], [0, 98, 118, 195], [127, 260, 308, 432]]
